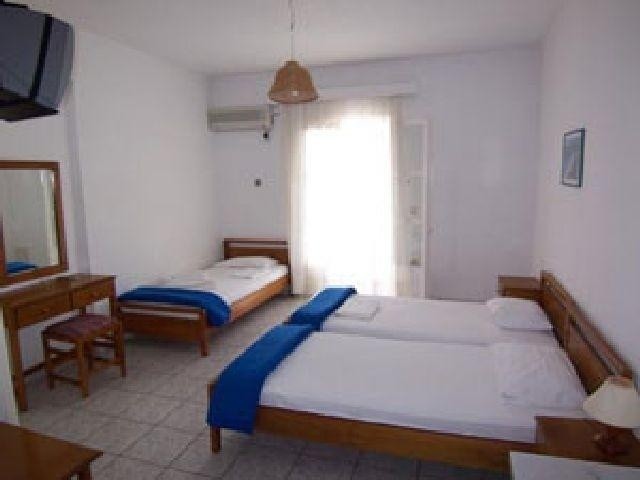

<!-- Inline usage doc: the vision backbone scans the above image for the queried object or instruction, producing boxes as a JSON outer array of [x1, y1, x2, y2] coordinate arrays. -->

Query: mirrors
[[0, 160, 68, 290]]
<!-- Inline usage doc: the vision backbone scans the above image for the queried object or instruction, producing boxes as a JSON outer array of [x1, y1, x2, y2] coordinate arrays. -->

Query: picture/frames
[[559, 126, 586, 188]]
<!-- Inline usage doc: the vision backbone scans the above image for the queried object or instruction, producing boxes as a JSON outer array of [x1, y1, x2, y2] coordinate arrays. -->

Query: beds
[[280, 268, 579, 348], [204, 306, 634, 475], [114, 236, 291, 358]]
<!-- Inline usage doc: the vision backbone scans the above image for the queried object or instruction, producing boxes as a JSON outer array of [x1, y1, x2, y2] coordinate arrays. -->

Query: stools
[[40, 313, 126, 399]]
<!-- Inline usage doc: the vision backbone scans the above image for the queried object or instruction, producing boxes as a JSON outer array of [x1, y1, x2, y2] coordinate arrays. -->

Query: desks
[[0, 272, 117, 411], [0, 420, 106, 480]]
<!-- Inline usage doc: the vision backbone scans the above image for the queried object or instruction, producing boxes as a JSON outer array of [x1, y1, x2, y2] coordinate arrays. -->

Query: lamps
[[267, 0, 321, 105], [582, 375, 640, 455]]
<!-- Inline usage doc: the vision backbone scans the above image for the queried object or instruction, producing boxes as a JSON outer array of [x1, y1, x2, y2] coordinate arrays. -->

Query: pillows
[[494, 340, 590, 410], [210, 255, 280, 269], [486, 297, 556, 332]]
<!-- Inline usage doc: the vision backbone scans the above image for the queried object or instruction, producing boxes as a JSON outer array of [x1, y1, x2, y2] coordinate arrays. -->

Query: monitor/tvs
[[0, 0, 73, 121]]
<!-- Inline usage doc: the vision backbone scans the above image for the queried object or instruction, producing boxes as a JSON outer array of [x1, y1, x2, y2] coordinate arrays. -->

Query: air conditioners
[[205, 104, 274, 134]]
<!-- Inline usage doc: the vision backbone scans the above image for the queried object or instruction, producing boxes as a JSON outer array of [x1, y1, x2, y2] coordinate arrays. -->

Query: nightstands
[[496, 273, 541, 299], [533, 412, 640, 468]]
